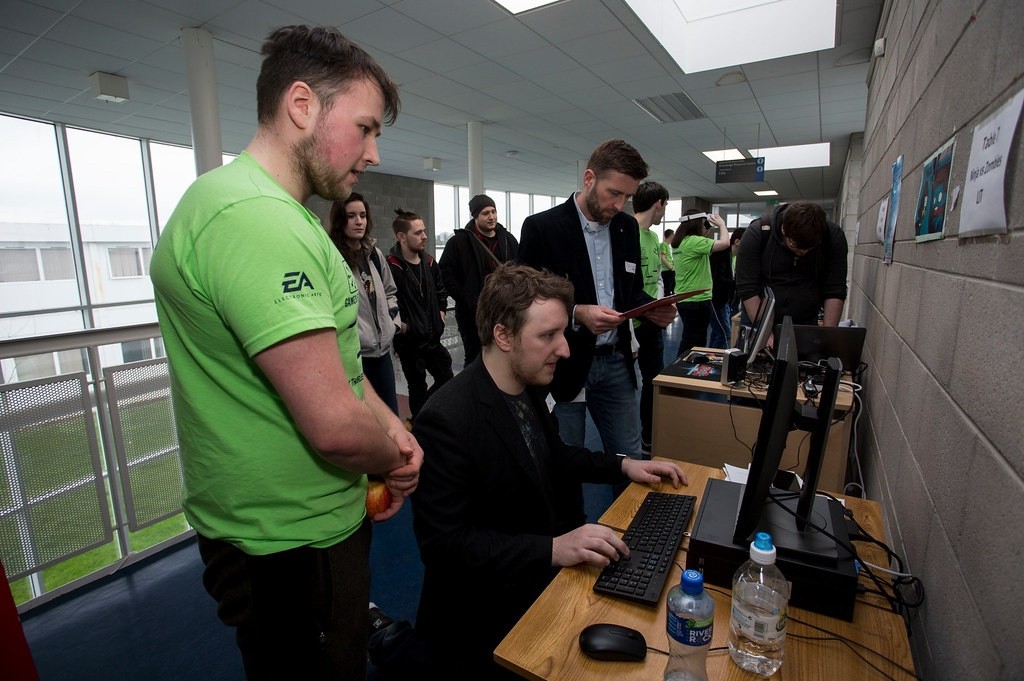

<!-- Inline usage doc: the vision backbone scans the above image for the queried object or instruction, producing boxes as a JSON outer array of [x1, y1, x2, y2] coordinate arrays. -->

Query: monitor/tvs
[[747, 286, 776, 364], [730, 314, 840, 561]]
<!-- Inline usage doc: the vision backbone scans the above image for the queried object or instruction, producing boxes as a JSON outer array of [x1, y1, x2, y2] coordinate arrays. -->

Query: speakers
[[721, 348, 747, 387]]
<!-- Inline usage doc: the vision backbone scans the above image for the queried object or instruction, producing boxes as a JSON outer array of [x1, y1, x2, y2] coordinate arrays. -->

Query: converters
[[802, 381, 818, 398]]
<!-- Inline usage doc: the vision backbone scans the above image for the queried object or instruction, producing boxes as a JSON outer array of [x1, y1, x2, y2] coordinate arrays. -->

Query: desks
[[493, 311, 917, 681]]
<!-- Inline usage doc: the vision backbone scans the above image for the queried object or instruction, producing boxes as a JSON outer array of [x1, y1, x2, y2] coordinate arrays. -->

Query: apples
[[366, 480, 391, 516]]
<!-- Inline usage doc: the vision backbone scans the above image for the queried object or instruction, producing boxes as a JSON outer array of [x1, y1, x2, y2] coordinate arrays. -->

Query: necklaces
[[404, 260, 423, 297]]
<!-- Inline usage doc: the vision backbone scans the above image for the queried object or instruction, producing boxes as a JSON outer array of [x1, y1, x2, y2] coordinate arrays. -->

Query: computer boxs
[[687, 478, 858, 623]]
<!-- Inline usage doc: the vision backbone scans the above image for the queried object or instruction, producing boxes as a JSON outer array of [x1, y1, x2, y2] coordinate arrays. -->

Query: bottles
[[663, 569, 715, 681], [726, 532, 789, 676]]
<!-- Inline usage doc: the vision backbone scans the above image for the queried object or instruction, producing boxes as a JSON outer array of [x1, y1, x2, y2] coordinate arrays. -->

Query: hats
[[469, 194, 495, 218]]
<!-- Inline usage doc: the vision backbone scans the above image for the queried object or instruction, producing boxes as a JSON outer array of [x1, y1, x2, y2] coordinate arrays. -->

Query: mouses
[[688, 353, 710, 364], [580, 623, 648, 663]]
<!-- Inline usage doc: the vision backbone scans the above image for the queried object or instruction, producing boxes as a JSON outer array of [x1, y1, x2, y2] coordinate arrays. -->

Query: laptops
[[775, 323, 868, 373]]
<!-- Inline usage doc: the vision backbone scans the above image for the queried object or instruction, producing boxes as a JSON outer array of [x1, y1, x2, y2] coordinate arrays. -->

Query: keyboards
[[593, 491, 697, 607]]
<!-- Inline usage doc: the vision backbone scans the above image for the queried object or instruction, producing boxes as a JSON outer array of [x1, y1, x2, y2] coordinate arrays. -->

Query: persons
[[518, 138, 676, 459], [734, 200, 848, 350], [326, 192, 401, 417], [382, 205, 455, 415], [670, 208, 760, 359], [632, 179, 669, 455], [660, 228, 676, 296], [150, 24, 423, 681], [439, 195, 521, 370], [412, 259, 688, 681]]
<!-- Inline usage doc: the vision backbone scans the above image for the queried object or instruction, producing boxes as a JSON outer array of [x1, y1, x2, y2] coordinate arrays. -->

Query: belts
[[594, 343, 621, 355]]
